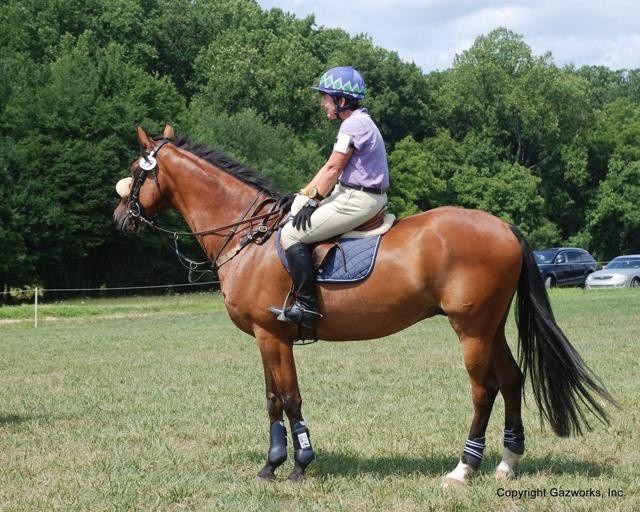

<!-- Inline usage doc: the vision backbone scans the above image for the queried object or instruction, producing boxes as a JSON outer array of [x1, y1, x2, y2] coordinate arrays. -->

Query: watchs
[[308, 187, 325, 202]]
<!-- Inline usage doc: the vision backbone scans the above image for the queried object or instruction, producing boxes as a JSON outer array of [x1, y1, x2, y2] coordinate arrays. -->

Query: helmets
[[309, 66, 366, 100]]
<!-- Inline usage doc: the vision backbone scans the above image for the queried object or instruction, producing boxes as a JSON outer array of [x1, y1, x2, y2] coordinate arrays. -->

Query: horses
[[113, 121, 626, 492]]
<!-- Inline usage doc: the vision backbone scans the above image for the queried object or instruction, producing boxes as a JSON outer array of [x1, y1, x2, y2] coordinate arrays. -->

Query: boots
[[266, 242, 325, 325]]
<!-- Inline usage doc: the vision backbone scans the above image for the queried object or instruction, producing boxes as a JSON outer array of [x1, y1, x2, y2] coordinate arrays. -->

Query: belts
[[339, 180, 384, 196]]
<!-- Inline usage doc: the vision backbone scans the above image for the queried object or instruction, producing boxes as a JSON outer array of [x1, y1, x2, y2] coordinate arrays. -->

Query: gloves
[[291, 198, 320, 232]]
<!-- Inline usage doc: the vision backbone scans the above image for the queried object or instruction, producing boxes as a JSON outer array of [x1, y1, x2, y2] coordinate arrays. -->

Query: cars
[[530, 246, 600, 291], [584, 254, 640, 290]]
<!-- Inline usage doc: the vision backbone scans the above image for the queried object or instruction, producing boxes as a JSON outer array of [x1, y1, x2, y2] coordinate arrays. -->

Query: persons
[[267, 65, 389, 330]]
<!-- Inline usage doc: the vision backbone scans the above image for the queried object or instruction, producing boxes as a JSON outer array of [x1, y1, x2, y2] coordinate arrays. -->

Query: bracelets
[[300, 189, 308, 195]]
